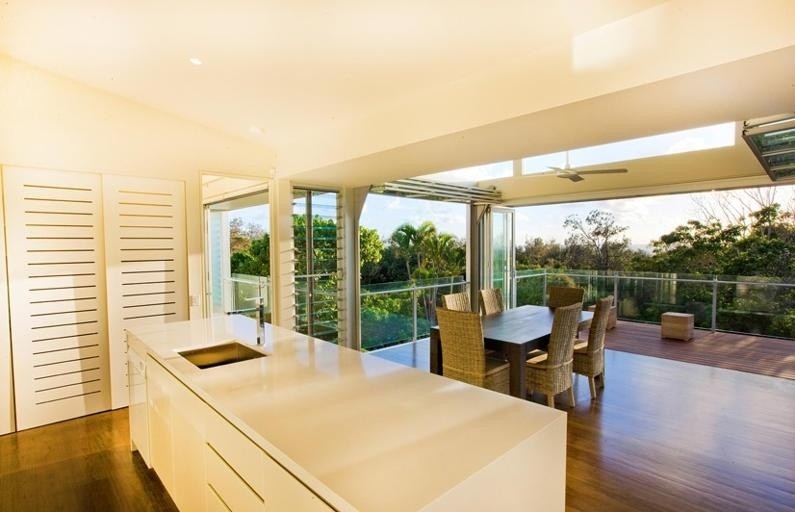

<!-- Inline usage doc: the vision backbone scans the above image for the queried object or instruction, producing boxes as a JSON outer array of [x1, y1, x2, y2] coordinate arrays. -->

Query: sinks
[[177, 343, 267, 369]]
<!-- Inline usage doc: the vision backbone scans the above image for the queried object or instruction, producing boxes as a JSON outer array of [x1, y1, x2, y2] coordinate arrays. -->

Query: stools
[[588, 304, 619, 332], [659, 311, 696, 342]]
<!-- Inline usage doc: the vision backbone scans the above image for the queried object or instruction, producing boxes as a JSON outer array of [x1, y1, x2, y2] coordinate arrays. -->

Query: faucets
[[226, 297, 264, 348]]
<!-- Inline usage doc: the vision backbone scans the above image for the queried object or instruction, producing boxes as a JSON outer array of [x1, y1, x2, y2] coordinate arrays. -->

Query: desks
[[429, 302, 601, 400]]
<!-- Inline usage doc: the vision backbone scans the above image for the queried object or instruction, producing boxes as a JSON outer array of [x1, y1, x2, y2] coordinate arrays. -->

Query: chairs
[[547, 284, 586, 312], [434, 306, 513, 396], [477, 288, 506, 316], [518, 302, 585, 410], [573, 295, 616, 402], [441, 291, 473, 313]]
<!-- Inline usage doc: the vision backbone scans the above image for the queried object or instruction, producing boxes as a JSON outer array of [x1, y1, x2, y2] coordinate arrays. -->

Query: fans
[[538, 151, 631, 183]]
